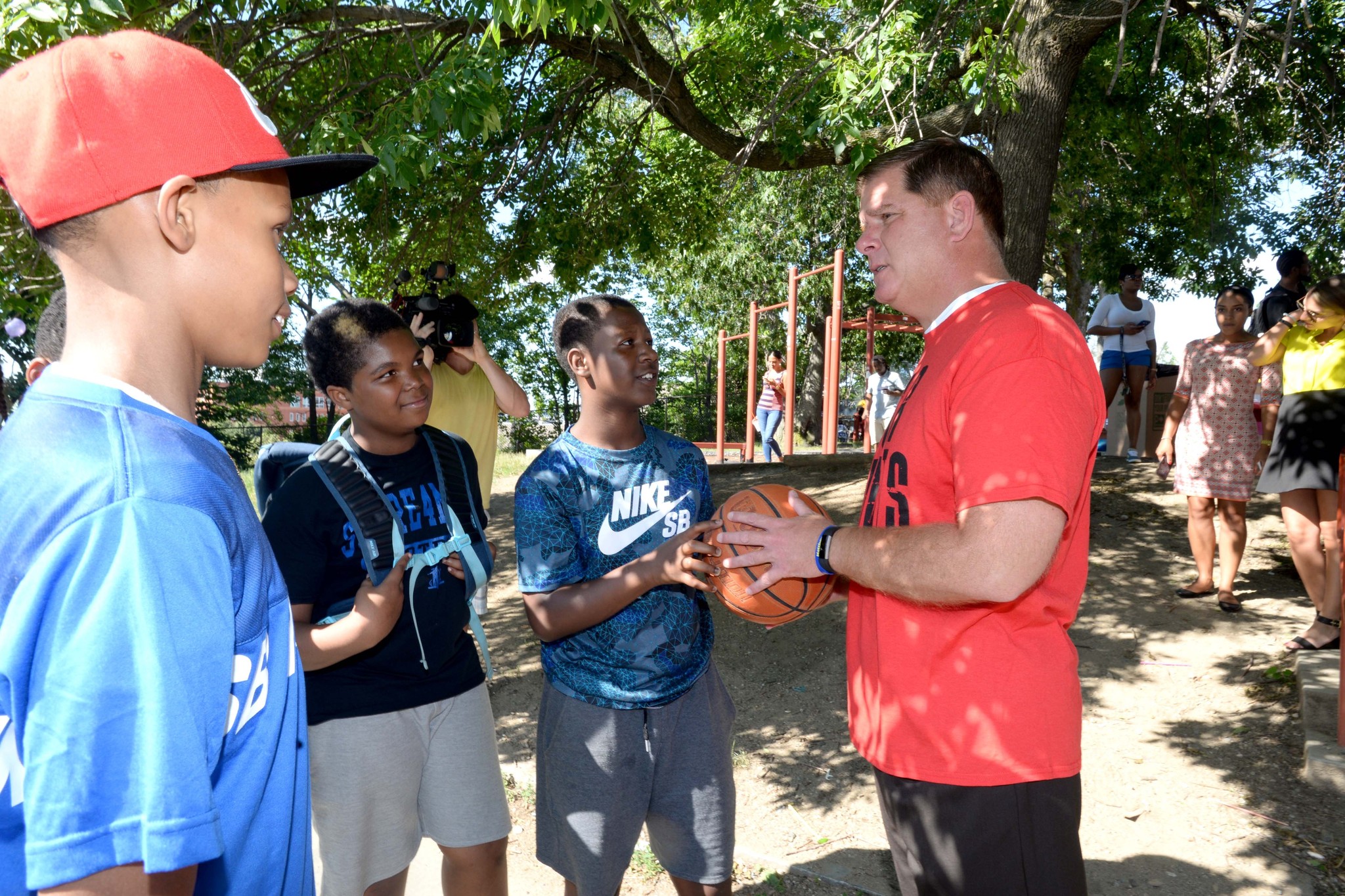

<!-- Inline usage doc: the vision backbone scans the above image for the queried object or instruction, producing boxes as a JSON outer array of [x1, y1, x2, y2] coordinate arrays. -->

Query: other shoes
[[1174, 582, 1214, 598], [1125, 448, 1141, 463], [1217, 591, 1241, 612]]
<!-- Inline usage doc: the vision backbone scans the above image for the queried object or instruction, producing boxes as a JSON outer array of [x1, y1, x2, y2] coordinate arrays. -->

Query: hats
[[0, 30, 379, 238]]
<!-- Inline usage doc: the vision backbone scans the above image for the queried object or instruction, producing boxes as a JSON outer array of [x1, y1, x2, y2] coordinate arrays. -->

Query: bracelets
[[1278, 318, 1293, 330], [1260, 439, 1272, 447], [814, 524, 838, 574], [1160, 436, 1173, 441], [1282, 312, 1297, 324], [1149, 368, 1157, 372]]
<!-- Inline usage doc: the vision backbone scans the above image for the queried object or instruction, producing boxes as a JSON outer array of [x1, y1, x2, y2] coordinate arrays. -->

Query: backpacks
[[1247, 288, 1288, 337], [254, 414, 491, 629]]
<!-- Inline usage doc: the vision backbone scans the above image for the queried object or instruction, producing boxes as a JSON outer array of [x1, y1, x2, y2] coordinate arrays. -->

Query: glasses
[[1124, 274, 1144, 281], [1296, 296, 1338, 323], [1297, 261, 1312, 269]]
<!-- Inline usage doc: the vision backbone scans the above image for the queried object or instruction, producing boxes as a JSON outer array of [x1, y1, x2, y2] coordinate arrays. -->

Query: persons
[[861, 354, 904, 453], [756, 349, 788, 462], [514, 295, 736, 895], [1156, 248, 1344, 649], [408, 297, 531, 527], [0, 26, 380, 895], [714, 136, 1105, 896], [264, 298, 511, 896], [1085, 266, 1157, 464]]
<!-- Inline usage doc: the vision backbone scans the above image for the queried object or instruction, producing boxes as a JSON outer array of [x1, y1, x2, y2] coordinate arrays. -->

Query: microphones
[[387, 270, 411, 292]]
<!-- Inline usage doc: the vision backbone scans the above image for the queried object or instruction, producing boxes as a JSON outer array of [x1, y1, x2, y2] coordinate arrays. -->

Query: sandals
[[1284, 615, 1341, 649]]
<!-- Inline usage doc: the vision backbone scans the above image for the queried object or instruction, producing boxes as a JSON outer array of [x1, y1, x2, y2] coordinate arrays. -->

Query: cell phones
[[877, 386, 888, 391], [1136, 320, 1151, 330], [1155, 455, 1175, 481], [762, 376, 769, 381]]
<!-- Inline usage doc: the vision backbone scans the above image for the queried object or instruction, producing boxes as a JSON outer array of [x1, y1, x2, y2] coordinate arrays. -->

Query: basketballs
[[702, 484, 834, 626]]
